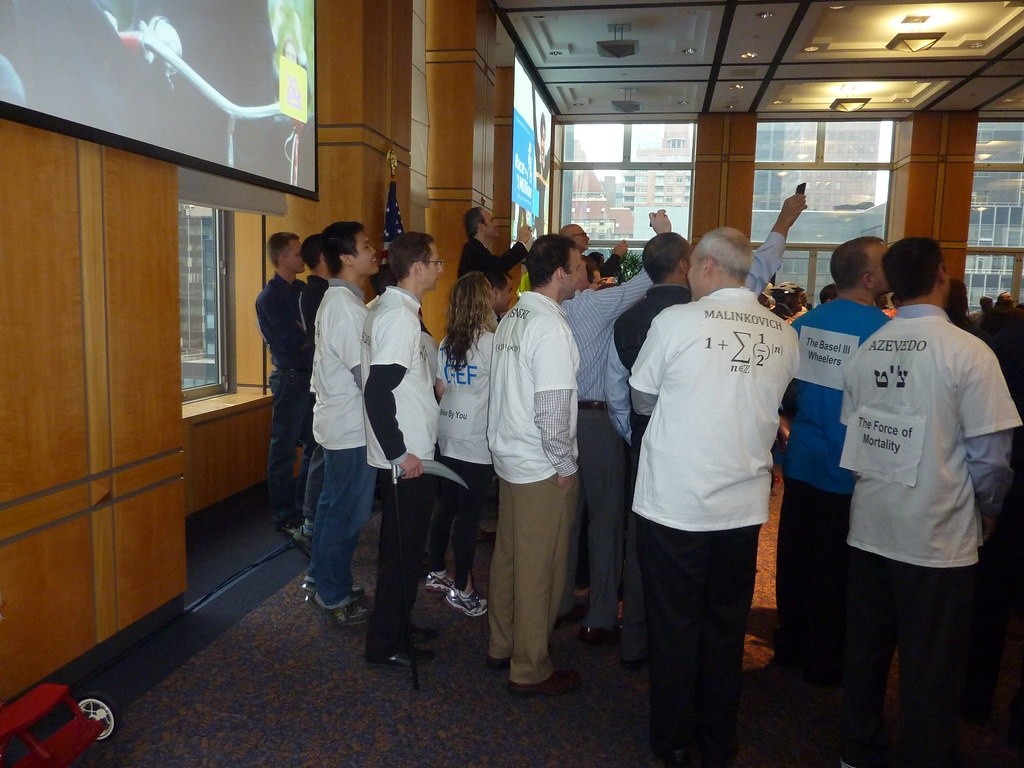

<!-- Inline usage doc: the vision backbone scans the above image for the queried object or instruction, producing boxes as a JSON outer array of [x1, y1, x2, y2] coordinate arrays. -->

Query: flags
[[382, 181, 431, 336]]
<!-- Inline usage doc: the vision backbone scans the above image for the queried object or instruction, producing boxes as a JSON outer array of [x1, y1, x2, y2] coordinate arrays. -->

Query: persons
[[484, 267, 513, 313], [629, 226, 800, 768], [293, 233, 332, 559], [772, 235, 893, 685], [604, 193, 807, 678], [962, 316, 1024, 746], [487, 234, 582, 693], [360, 230, 440, 665], [557, 210, 671, 672], [270, 1, 312, 110], [458, 206, 532, 278], [256, 232, 305, 534], [426, 273, 494, 616], [304, 221, 379, 625], [532, 84, 550, 238], [840, 238, 1024, 768], [516, 224, 1024, 464]]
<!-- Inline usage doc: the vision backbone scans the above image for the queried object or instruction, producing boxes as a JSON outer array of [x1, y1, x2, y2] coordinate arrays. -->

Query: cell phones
[[796, 182, 807, 195]]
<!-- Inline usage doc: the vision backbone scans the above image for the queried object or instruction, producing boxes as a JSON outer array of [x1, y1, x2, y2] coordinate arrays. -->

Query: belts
[[577, 399, 607, 410], [272, 364, 309, 372]]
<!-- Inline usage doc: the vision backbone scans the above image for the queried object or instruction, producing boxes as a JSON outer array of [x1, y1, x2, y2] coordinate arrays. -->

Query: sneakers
[[444, 583, 488, 618], [305, 590, 371, 627], [425, 570, 455, 593], [275, 514, 304, 533], [292, 525, 314, 557], [302, 579, 365, 604]]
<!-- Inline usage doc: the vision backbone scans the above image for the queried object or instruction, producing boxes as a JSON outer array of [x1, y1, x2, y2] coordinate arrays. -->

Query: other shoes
[[659, 746, 690, 768], [700, 752, 733, 768]]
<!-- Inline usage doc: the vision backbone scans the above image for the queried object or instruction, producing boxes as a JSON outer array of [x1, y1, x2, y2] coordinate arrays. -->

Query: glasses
[[489, 218, 496, 223], [572, 232, 586, 238], [409, 258, 444, 267]]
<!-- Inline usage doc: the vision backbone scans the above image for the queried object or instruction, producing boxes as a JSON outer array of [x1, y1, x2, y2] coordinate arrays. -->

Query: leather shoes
[[618, 656, 649, 673], [508, 669, 582, 697], [413, 624, 440, 643], [486, 653, 511, 671], [577, 621, 621, 645], [368, 644, 436, 667]]
